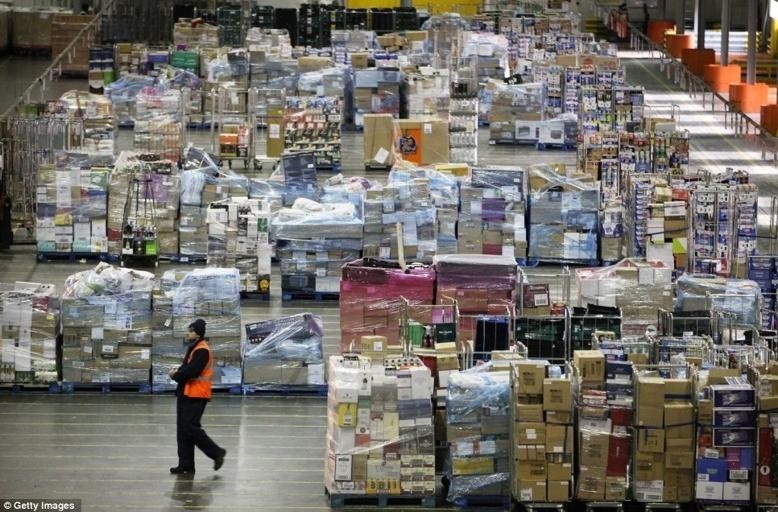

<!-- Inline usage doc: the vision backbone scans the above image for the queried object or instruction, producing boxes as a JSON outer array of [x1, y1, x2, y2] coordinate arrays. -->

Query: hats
[[190, 319, 206, 337]]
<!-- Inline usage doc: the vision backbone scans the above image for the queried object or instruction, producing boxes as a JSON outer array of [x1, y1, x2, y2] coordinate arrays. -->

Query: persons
[[169, 318, 226, 475], [170, 474, 224, 512]]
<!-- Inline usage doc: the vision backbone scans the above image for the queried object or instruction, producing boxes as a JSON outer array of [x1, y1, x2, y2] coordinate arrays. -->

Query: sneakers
[[214, 449, 225, 470], [170, 466, 195, 474]]
